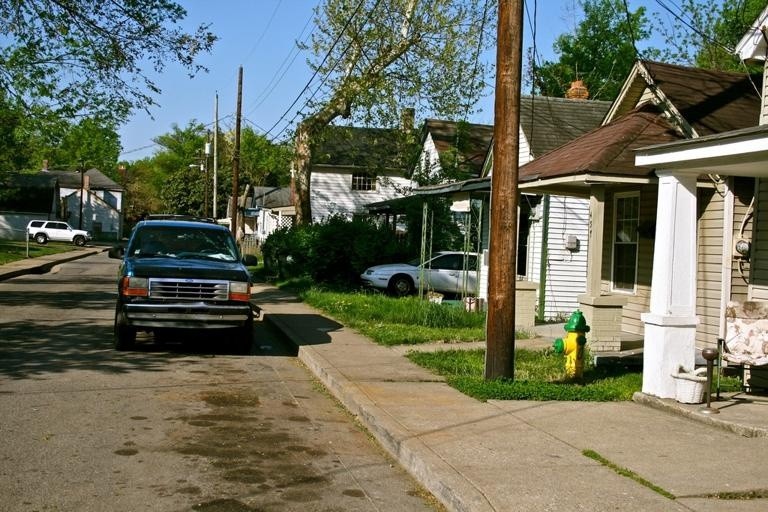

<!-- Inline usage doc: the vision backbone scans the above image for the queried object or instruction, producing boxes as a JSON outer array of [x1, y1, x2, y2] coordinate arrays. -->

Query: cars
[[360, 250, 480, 297]]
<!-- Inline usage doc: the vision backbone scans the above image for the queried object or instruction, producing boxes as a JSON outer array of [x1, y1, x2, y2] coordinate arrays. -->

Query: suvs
[[24, 219, 92, 247], [107, 218, 257, 352]]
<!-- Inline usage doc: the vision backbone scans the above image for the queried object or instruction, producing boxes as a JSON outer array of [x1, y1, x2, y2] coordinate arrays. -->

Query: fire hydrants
[[552, 308, 588, 381]]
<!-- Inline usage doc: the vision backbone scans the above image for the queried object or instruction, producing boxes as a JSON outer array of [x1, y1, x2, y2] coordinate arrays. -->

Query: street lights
[[189, 162, 208, 222]]
